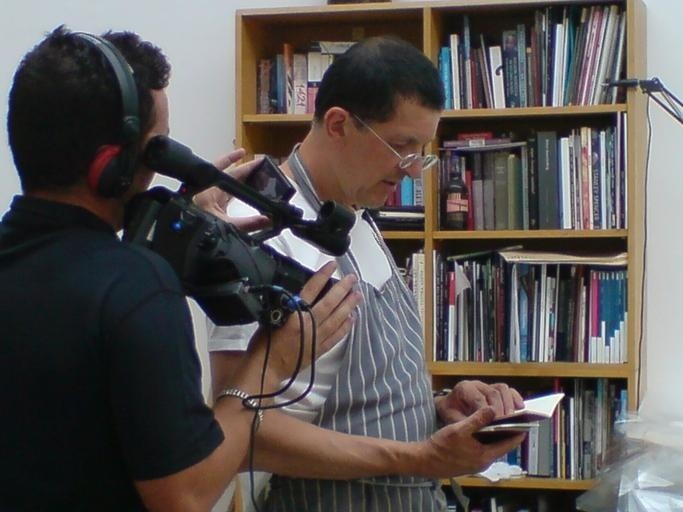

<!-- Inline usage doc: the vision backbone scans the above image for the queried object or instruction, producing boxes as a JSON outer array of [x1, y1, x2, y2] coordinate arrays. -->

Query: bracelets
[[215, 389, 263, 432]]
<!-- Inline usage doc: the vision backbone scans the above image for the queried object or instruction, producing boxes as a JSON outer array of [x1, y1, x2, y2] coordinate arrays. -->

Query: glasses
[[352, 112, 438, 171]]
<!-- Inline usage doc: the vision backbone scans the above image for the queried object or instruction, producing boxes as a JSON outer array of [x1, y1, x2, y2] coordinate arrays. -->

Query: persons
[[0, 24, 361, 512], [206, 37, 525, 512]]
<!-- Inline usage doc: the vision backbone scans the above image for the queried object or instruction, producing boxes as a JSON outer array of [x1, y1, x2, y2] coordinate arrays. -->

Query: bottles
[[445, 172, 467, 230]]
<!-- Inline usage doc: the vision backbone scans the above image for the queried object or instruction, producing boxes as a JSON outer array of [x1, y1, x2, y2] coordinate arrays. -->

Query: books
[[437, 111, 627, 232], [255, 154, 287, 167], [386, 172, 424, 212], [447, 489, 547, 511], [396, 248, 424, 337], [438, 4, 627, 111], [432, 245, 627, 364], [490, 376, 627, 481], [259, 40, 356, 115]]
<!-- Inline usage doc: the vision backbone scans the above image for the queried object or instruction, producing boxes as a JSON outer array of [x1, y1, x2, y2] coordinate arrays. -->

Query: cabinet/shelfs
[[236, 3, 649, 488]]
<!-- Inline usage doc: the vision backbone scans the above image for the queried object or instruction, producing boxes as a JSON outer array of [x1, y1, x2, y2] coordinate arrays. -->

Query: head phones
[[74, 29, 147, 202]]
